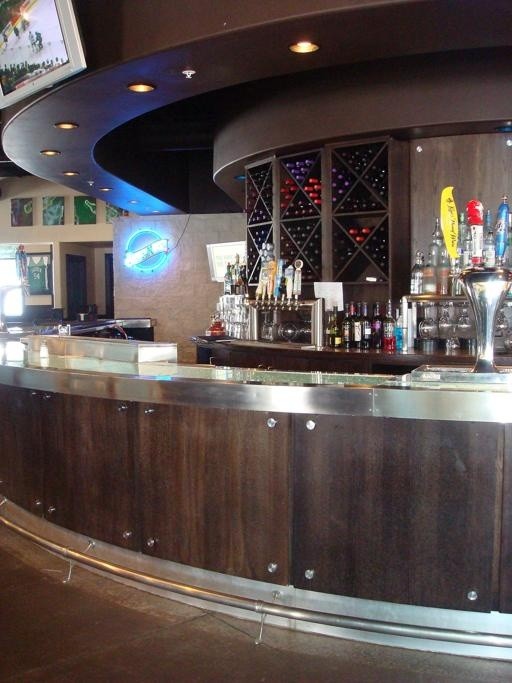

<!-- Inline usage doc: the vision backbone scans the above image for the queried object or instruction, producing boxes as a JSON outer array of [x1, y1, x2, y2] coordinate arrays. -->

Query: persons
[[14, 26, 20, 40], [23, 18, 29, 26], [0, 57, 64, 95], [21, 20, 25, 30], [3, 34, 8, 48], [28, 31, 37, 52], [35, 32, 43, 49]]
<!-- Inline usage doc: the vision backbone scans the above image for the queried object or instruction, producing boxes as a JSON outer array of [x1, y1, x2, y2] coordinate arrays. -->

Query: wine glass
[[419, 300, 506, 341], [260, 310, 275, 343]]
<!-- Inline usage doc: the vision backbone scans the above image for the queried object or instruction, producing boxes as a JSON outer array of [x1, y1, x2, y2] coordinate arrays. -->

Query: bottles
[[247, 142, 390, 283], [223, 254, 249, 296], [59, 322, 69, 337], [409, 209, 512, 299], [40, 357, 50, 370], [39, 339, 50, 356], [326, 297, 410, 353]]
